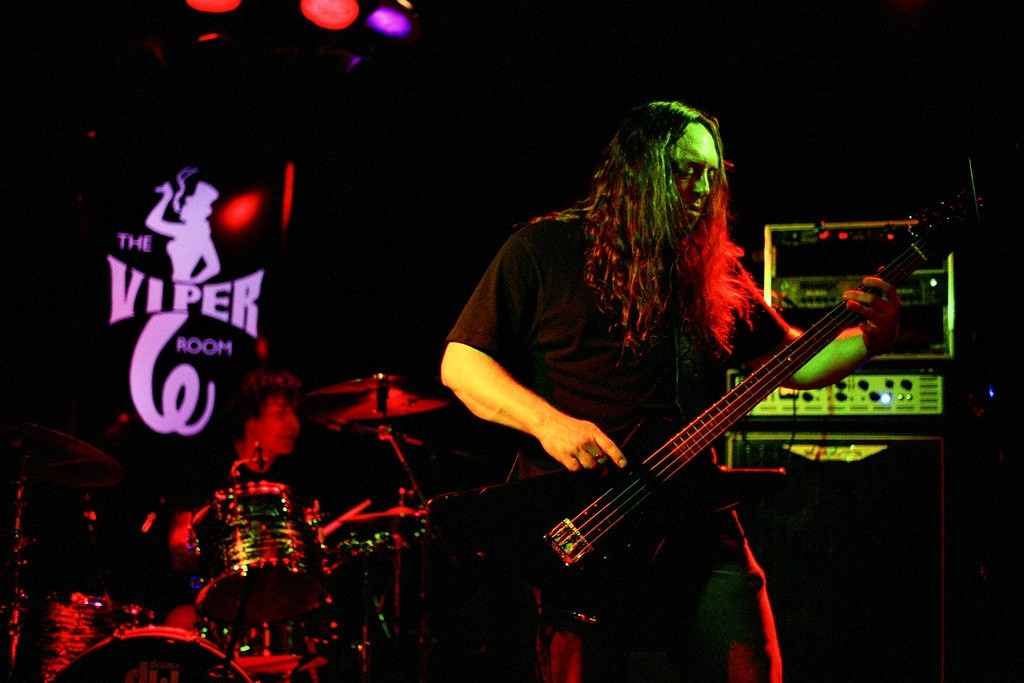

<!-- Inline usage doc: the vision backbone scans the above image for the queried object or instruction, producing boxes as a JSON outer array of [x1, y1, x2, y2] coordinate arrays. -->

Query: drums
[[184, 480, 328, 627], [51, 624, 255, 683]]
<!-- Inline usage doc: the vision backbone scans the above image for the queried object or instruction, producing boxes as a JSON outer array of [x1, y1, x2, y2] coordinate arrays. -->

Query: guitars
[[426, 189, 976, 614]]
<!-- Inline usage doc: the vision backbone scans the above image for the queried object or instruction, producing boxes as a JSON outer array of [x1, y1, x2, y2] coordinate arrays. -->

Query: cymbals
[[294, 372, 447, 431], [0, 416, 123, 490]]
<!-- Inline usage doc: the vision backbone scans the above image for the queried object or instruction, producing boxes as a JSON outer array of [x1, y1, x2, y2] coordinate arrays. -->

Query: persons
[[163, 369, 353, 682], [438, 98, 902, 683]]
